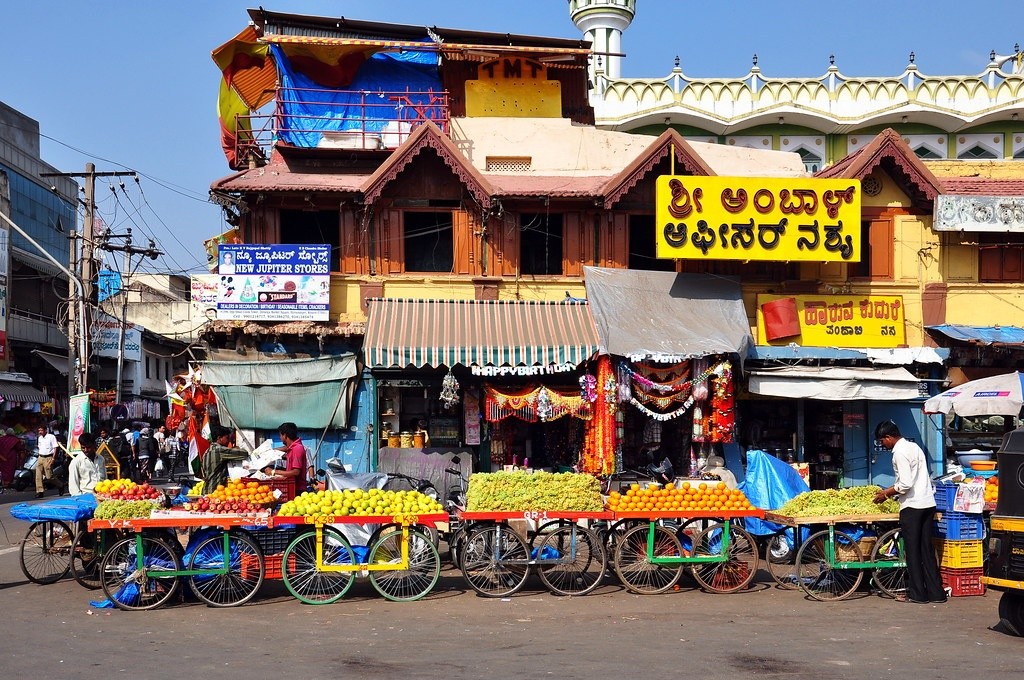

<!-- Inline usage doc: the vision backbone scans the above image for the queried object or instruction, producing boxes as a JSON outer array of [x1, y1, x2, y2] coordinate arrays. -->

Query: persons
[[71, 402, 88, 450], [0, 423, 190, 497], [68, 433, 108, 579], [872, 420, 948, 604], [265, 422, 315, 568], [220, 252, 235, 274], [202, 426, 248, 495]]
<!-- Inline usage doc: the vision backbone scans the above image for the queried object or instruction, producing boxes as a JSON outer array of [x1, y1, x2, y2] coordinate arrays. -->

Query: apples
[[100, 483, 160, 500], [186, 497, 266, 514]]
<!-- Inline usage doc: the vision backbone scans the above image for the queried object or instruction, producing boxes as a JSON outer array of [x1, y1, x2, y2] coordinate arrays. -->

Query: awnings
[[255, 32, 626, 71], [0, 379, 50, 402], [32, 349, 76, 377], [210, 25, 277, 113], [364, 296, 601, 368]]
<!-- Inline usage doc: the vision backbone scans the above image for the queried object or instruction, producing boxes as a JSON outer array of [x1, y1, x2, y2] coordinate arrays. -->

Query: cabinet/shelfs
[[378, 386, 398, 448]]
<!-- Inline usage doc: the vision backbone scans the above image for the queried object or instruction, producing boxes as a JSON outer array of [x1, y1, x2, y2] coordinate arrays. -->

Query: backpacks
[[119, 431, 132, 456]]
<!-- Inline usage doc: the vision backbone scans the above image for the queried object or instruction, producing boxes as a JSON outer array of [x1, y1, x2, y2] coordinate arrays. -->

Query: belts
[[39, 454, 53, 458]]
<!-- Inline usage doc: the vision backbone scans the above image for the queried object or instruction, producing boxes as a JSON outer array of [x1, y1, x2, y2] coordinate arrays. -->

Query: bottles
[[762, 448, 768, 454], [382, 397, 393, 414], [388, 431, 399, 448], [787, 449, 794, 462], [400, 431, 414, 448], [381, 422, 392, 438], [414, 431, 426, 448], [775, 449, 782, 460]]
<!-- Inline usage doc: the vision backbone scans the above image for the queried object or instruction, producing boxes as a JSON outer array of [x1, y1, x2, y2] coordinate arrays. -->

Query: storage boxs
[[240, 477, 296, 580], [932, 484, 984, 596]]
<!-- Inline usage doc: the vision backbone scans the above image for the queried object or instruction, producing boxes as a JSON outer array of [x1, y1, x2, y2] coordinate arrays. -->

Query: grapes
[[774, 485, 900, 518], [466, 469, 604, 512], [93, 499, 161, 520]]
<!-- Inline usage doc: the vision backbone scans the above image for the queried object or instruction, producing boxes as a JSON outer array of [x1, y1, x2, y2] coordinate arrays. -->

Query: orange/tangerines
[[207, 478, 275, 504], [673, 585, 680, 590], [605, 481, 756, 511], [963, 476, 998, 503]]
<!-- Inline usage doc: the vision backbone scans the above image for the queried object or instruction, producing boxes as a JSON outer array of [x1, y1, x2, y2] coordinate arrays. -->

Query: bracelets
[[270, 468, 276, 476]]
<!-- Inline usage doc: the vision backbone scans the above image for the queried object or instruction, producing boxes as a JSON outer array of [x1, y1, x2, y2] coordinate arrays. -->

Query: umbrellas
[[922, 371, 1024, 429]]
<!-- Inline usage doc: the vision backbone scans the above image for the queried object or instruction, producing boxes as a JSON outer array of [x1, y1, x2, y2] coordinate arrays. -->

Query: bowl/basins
[[969, 461, 998, 471], [955, 451, 994, 467], [162, 487, 182, 496]]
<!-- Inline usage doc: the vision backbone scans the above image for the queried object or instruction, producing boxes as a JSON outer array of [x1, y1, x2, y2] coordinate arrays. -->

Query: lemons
[[95, 478, 136, 493], [187, 483, 203, 496], [278, 488, 444, 516]]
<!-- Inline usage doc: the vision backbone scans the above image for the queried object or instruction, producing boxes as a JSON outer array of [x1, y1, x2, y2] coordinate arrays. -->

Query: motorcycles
[[978, 428, 1024, 637]]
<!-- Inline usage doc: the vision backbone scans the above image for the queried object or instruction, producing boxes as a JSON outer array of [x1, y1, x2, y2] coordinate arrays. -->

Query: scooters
[[15, 448, 65, 492]]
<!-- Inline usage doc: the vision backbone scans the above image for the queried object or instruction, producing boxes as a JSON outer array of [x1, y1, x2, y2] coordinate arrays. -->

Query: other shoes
[[930, 596, 948, 603], [36, 492, 44, 498], [59, 485, 64, 496], [168, 478, 175, 483], [896, 595, 929, 604]]
